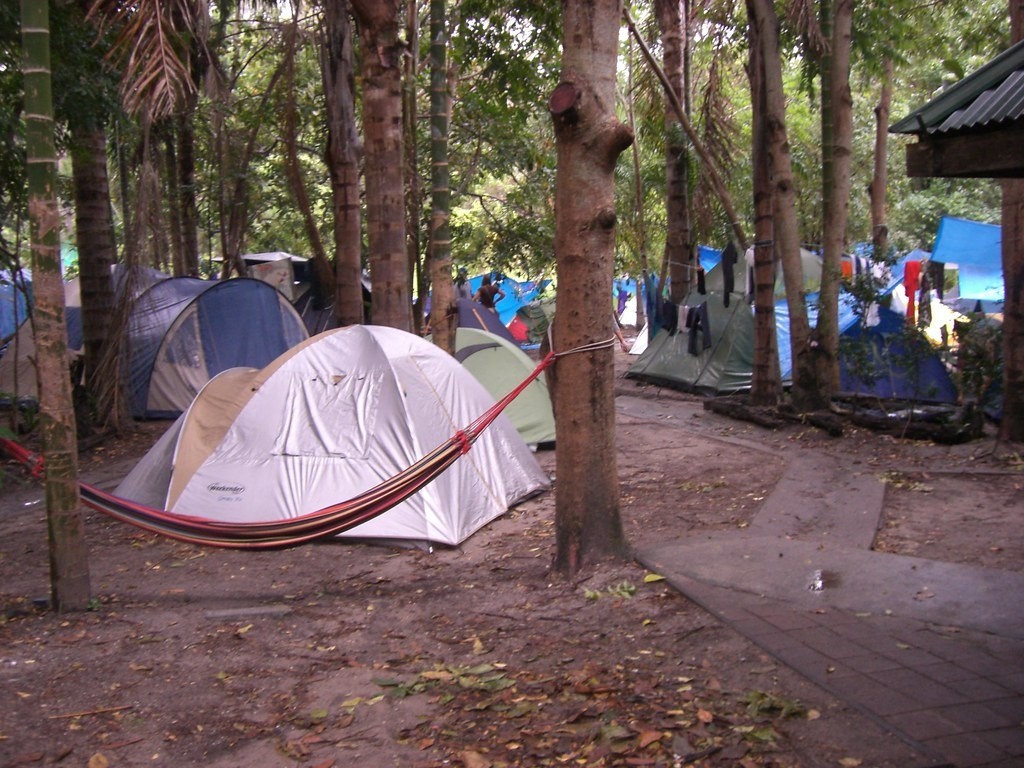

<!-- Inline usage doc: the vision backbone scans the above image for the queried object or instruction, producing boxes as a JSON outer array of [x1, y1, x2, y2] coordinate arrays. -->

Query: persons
[[473, 275, 505, 319], [455, 268, 471, 298]]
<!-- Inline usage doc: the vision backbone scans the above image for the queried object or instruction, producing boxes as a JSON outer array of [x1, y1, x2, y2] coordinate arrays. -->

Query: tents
[[110, 325, 552, 548], [1, 218, 1005, 451]]
